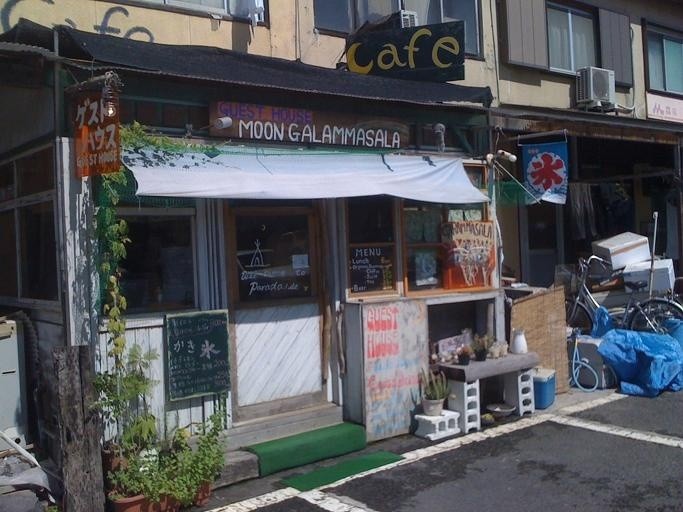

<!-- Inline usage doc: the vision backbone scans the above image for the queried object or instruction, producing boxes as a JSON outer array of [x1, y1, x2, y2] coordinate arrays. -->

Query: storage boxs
[[533, 367, 557, 409]]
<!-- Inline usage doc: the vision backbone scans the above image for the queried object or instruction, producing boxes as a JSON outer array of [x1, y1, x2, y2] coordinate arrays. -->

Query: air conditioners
[[576, 66, 616, 108], [392, 9, 420, 30]]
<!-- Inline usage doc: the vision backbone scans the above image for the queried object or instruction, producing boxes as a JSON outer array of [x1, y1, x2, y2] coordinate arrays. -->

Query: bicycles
[[566, 254, 683, 335]]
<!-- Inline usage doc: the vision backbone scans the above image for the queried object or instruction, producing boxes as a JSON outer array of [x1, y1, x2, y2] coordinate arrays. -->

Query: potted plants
[[89, 165, 222, 511], [457, 333, 498, 365], [417, 373, 449, 413]]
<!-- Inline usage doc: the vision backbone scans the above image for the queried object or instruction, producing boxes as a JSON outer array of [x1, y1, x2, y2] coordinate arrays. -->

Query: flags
[[523, 143, 569, 205]]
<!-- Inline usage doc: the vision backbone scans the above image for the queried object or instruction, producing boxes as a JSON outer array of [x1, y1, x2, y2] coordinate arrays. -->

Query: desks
[[436, 350, 540, 435]]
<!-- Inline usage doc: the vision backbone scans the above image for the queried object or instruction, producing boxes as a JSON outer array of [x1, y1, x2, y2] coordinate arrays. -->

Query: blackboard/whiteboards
[[223, 206, 401, 309], [166, 309, 233, 401]]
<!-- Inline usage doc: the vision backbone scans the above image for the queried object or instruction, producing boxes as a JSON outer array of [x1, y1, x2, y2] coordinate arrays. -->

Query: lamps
[[196, 116, 233, 136], [489, 149, 516, 166]]
